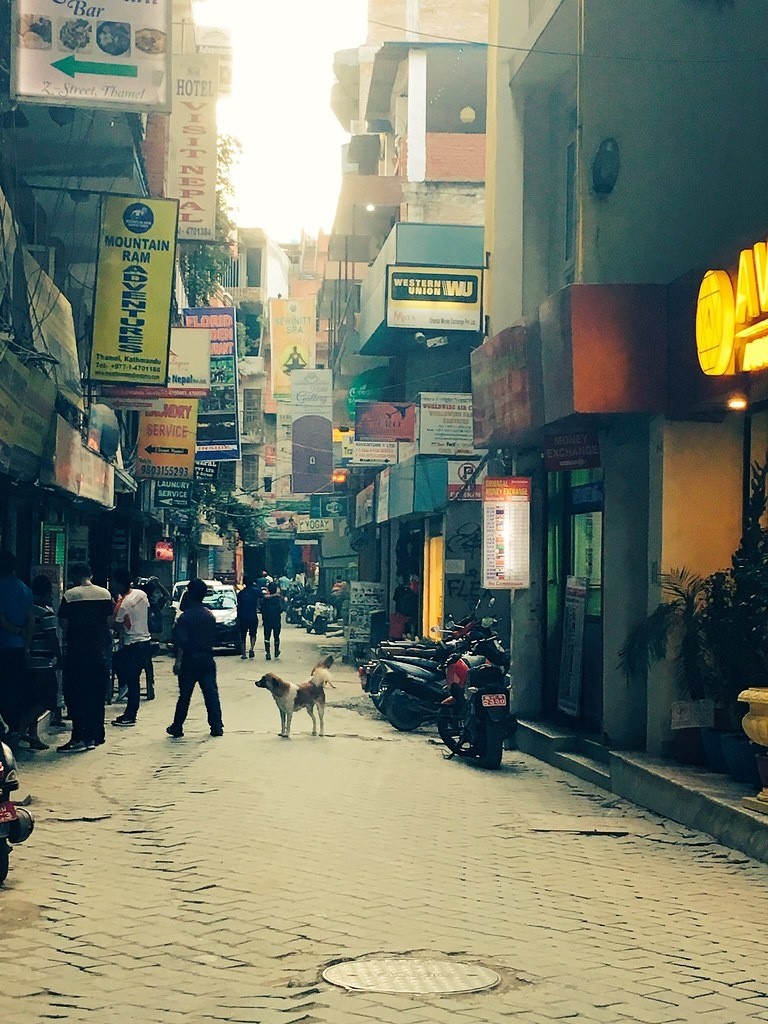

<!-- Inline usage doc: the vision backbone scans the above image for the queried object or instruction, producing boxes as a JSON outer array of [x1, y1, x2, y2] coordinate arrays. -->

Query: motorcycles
[[356, 599, 519, 770], [285, 584, 338, 635], [0, 713, 38, 894]]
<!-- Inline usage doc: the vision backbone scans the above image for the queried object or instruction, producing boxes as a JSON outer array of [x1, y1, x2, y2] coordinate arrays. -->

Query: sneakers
[[210, 726, 224, 737], [116, 685, 128, 702], [57, 739, 103, 753], [112, 715, 137, 726], [148, 692, 155, 700], [10, 732, 48, 749], [167, 724, 183, 737]]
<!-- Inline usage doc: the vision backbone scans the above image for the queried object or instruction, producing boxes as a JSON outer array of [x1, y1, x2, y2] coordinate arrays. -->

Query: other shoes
[[266, 653, 271, 660], [241, 650, 254, 659], [275, 651, 280, 657]]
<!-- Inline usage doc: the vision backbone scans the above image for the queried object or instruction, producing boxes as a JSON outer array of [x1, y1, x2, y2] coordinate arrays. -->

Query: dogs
[[254, 665, 334, 739]]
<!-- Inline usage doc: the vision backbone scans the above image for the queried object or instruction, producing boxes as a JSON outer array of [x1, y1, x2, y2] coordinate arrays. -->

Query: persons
[[237, 570, 346, 660], [167, 579, 224, 736], [0, 551, 156, 753]]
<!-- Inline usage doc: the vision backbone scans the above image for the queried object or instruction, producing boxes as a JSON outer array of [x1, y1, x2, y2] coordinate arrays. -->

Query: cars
[[171, 576, 243, 649]]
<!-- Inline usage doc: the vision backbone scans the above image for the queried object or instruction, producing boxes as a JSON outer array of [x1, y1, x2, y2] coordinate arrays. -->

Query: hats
[[243, 576, 255, 584]]
